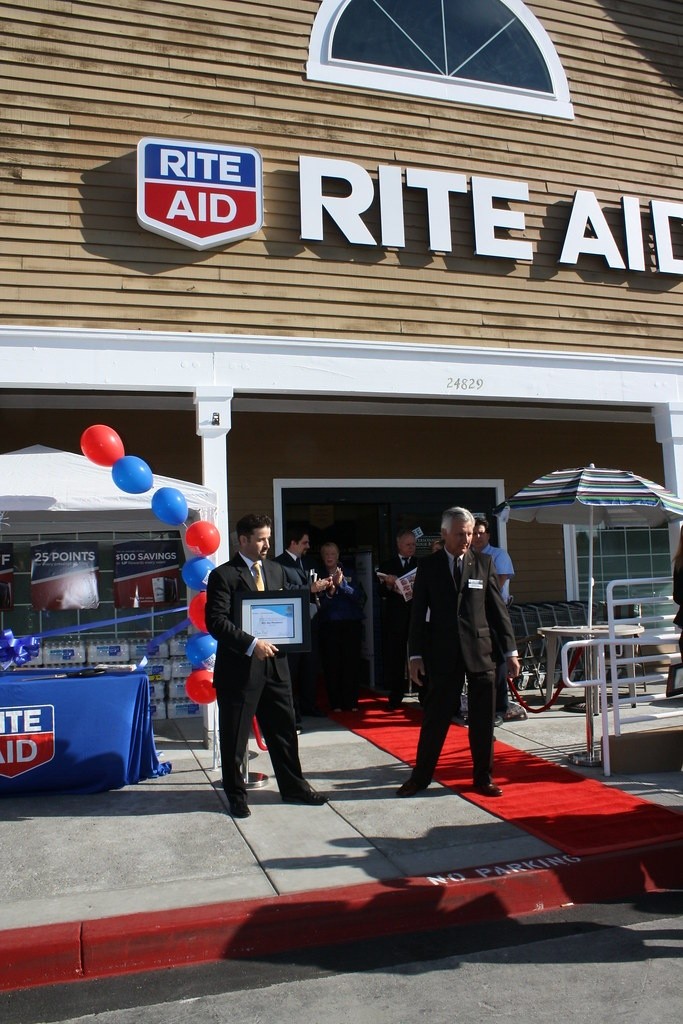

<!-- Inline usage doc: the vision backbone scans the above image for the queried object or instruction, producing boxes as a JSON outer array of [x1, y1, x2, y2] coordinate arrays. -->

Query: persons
[[459, 517, 515, 727], [272, 525, 368, 734], [396, 506, 521, 798], [673, 526, 683, 665], [431, 539, 444, 554], [205, 512, 333, 818], [376, 528, 425, 709]]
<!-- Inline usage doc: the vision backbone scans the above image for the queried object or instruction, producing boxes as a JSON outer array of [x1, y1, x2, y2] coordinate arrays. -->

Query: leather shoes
[[228, 799, 251, 817], [493, 713, 502, 727], [477, 783, 502, 795], [396, 778, 429, 796], [282, 791, 330, 806]]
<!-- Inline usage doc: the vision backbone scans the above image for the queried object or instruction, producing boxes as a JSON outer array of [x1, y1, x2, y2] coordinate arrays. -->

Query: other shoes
[[302, 707, 328, 718]]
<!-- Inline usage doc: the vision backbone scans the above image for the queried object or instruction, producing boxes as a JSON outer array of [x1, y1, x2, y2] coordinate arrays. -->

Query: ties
[[252, 563, 265, 593], [296, 557, 303, 570], [403, 557, 408, 568]]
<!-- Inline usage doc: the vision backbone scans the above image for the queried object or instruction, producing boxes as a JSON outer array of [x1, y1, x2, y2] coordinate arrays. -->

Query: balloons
[[151, 487, 188, 526], [189, 592, 211, 634], [186, 520, 220, 556], [183, 631, 219, 667], [113, 455, 154, 494], [83, 426, 124, 466], [186, 670, 217, 703], [183, 557, 216, 590]]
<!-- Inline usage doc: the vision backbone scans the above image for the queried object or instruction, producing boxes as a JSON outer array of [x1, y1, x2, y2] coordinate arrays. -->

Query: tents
[[0, 442, 222, 772]]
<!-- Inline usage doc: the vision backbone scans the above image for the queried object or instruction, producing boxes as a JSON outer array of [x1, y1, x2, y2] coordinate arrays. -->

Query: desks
[[0, 666, 172, 800], [537, 624, 645, 709]]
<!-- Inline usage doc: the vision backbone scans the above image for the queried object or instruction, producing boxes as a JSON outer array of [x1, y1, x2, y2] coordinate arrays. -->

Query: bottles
[[11, 636, 198, 704]]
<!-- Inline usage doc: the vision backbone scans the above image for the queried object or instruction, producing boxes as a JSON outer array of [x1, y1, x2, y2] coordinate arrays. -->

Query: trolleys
[[459, 600, 608, 727]]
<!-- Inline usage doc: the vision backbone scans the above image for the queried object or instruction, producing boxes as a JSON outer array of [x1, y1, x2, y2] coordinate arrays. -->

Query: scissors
[[21, 667, 107, 681]]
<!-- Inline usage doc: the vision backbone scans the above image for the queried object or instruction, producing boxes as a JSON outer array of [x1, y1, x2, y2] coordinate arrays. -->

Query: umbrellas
[[490, 463, 683, 627]]
